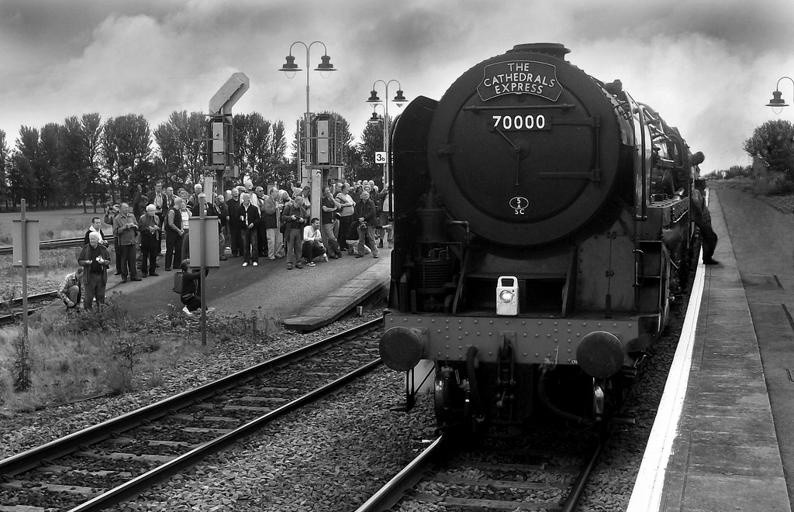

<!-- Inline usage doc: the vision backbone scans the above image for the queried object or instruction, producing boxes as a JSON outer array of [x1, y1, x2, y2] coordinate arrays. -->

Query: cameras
[[295, 216, 300, 221], [103, 241, 109, 245], [108, 205, 113, 210]]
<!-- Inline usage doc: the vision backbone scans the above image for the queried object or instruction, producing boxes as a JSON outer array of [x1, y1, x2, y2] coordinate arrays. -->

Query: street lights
[[371, 103, 386, 190], [766, 77, 794, 115], [281, 41, 332, 169], [367, 79, 406, 210]]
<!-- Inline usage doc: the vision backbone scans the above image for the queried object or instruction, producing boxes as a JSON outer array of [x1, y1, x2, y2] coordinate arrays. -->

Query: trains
[[380, 41, 702, 458]]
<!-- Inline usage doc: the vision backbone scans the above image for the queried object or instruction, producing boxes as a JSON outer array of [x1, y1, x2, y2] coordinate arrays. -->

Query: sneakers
[[253, 262, 258, 266], [183, 307, 192, 314], [287, 262, 316, 269], [241, 262, 247, 266], [114, 270, 159, 283], [165, 267, 171, 271], [173, 266, 180, 269]]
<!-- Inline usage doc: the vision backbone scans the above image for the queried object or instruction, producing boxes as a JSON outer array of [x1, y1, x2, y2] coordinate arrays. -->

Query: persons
[[77, 217, 111, 307], [61, 267, 84, 307], [692, 177, 719, 265], [104, 175, 392, 284]]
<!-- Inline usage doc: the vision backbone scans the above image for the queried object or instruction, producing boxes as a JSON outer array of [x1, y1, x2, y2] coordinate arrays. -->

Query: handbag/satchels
[[173, 272, 183, 294]]
